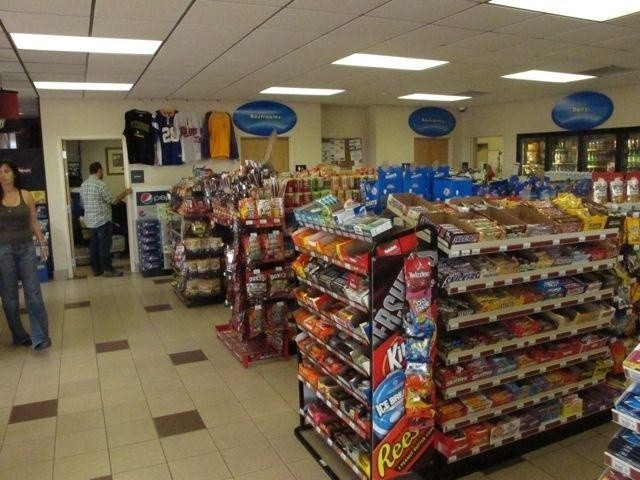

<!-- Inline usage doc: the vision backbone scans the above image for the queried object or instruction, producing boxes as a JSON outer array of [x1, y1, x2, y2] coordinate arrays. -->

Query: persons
[[79, 162, 133, 277], [0, 158, 51, 350]]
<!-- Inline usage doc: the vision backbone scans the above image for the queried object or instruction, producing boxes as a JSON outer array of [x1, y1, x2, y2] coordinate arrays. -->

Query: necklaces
[[2, 192, 17, 213]]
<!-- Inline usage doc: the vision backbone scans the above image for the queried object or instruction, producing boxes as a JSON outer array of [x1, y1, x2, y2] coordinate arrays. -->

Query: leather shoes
[[25, 339, 50, 352]]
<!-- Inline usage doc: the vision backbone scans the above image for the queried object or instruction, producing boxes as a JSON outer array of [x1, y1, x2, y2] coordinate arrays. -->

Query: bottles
[[550, 135, 639, 172]]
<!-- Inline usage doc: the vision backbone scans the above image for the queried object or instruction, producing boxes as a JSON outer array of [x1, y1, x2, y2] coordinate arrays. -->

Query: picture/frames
[[105, 147, 124, 176]]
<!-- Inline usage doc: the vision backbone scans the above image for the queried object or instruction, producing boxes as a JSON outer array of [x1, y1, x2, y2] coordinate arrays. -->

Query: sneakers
[[94, 270, 122, 277]]
